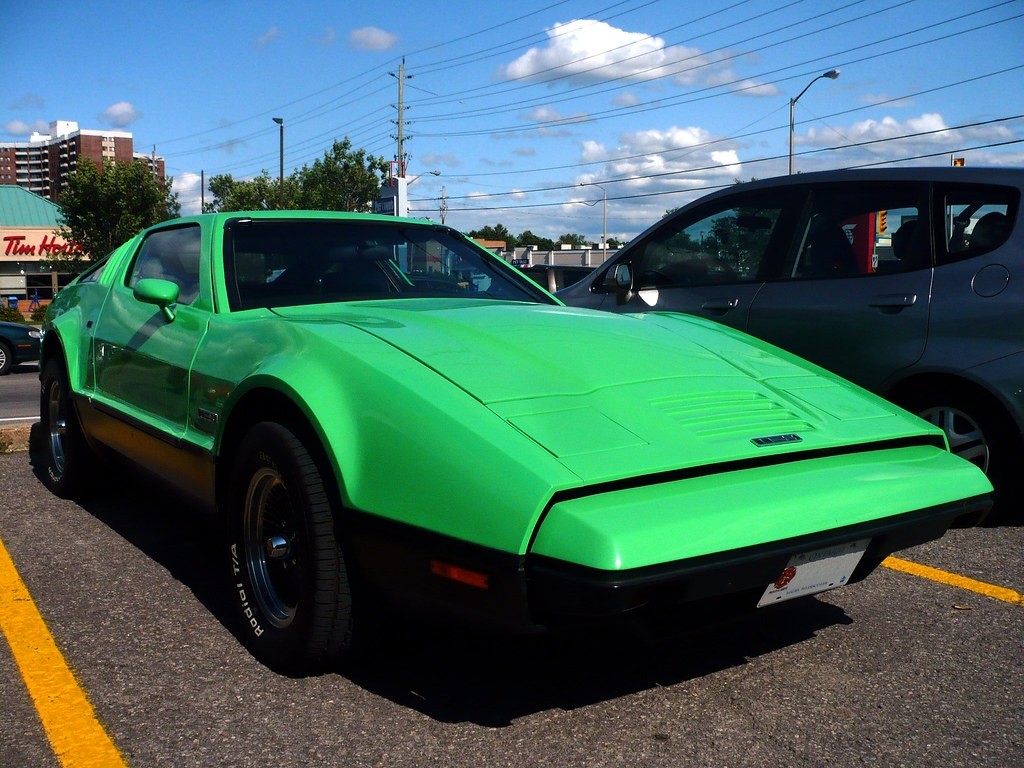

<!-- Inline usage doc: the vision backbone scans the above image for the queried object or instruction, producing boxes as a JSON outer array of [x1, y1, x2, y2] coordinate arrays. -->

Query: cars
[[0, 321, 43, 374], [549, 166, 1023, 526]]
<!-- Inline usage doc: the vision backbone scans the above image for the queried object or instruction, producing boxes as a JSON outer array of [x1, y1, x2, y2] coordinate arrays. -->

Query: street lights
[[788, 69, 841, 176], [272, 118, 285, 210], [580, 182, 607, 264]]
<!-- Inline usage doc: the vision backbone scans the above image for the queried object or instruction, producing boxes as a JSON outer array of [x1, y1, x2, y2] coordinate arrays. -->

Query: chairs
[[800, 228, 858, 277], [877, 220, 930, 272], [943, 212, 1007, 262]]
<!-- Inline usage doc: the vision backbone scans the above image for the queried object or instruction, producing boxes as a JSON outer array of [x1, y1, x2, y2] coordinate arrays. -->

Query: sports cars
[[35, 207, 996, 677]]
